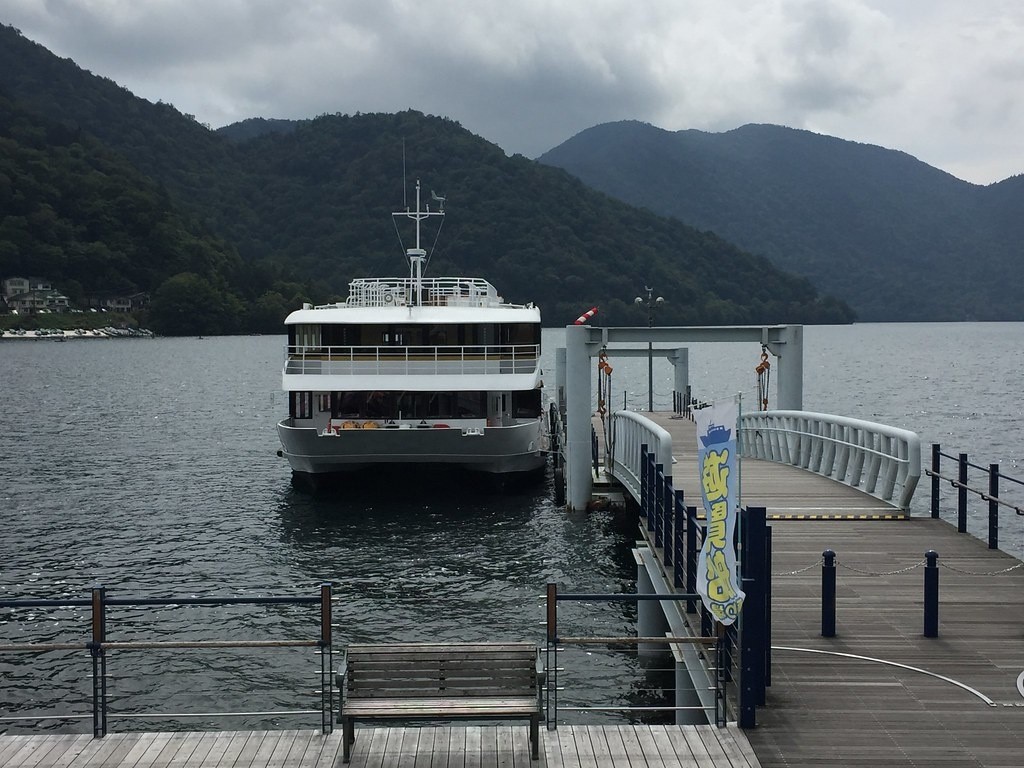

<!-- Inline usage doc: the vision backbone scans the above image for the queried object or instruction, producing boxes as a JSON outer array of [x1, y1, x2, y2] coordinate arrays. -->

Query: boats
[[275, 138, 551, 490]]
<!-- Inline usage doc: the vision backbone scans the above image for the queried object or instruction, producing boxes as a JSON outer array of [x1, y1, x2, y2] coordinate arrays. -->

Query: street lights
[[634, 286, 665, 411]]
